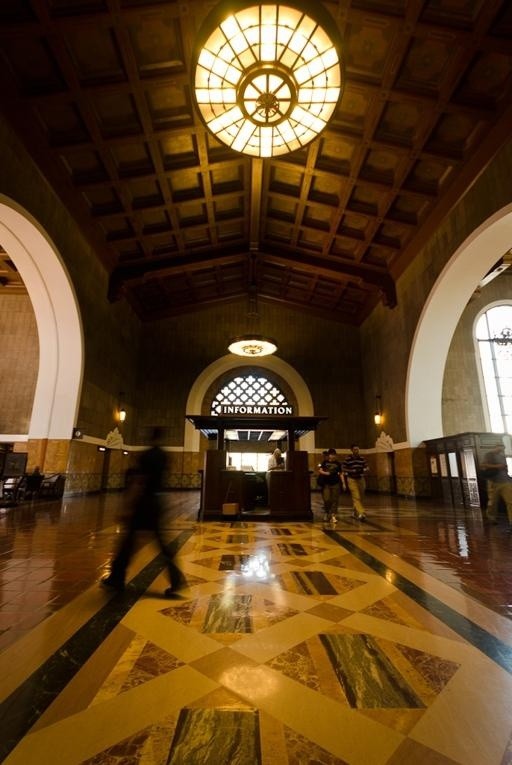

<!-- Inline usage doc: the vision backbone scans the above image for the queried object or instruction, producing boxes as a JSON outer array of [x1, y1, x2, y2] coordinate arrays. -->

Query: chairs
[[2, 473, 65, 505]]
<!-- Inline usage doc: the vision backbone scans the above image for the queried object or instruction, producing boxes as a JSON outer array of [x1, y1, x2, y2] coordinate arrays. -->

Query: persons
[[317, 451, 328, 511], [478, 442, 512, 534], [341, 446, 370, 523], [98, 425, 188, 599], [317, 449, 346, 524], [265, 448, 283, 506]]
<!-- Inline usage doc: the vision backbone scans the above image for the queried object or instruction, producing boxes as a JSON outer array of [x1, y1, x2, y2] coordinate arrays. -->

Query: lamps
[[190, 0, 344, 159], [374, 394, 381, 426], [119, 391, 126, 423], [122, 451, 129, 456], [98, 446, 106, 452], [225, 254, 279, 358]]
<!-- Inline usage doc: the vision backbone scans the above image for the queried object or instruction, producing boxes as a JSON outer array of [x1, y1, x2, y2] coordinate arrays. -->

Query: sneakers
[[322, 511, 369, 523]]
